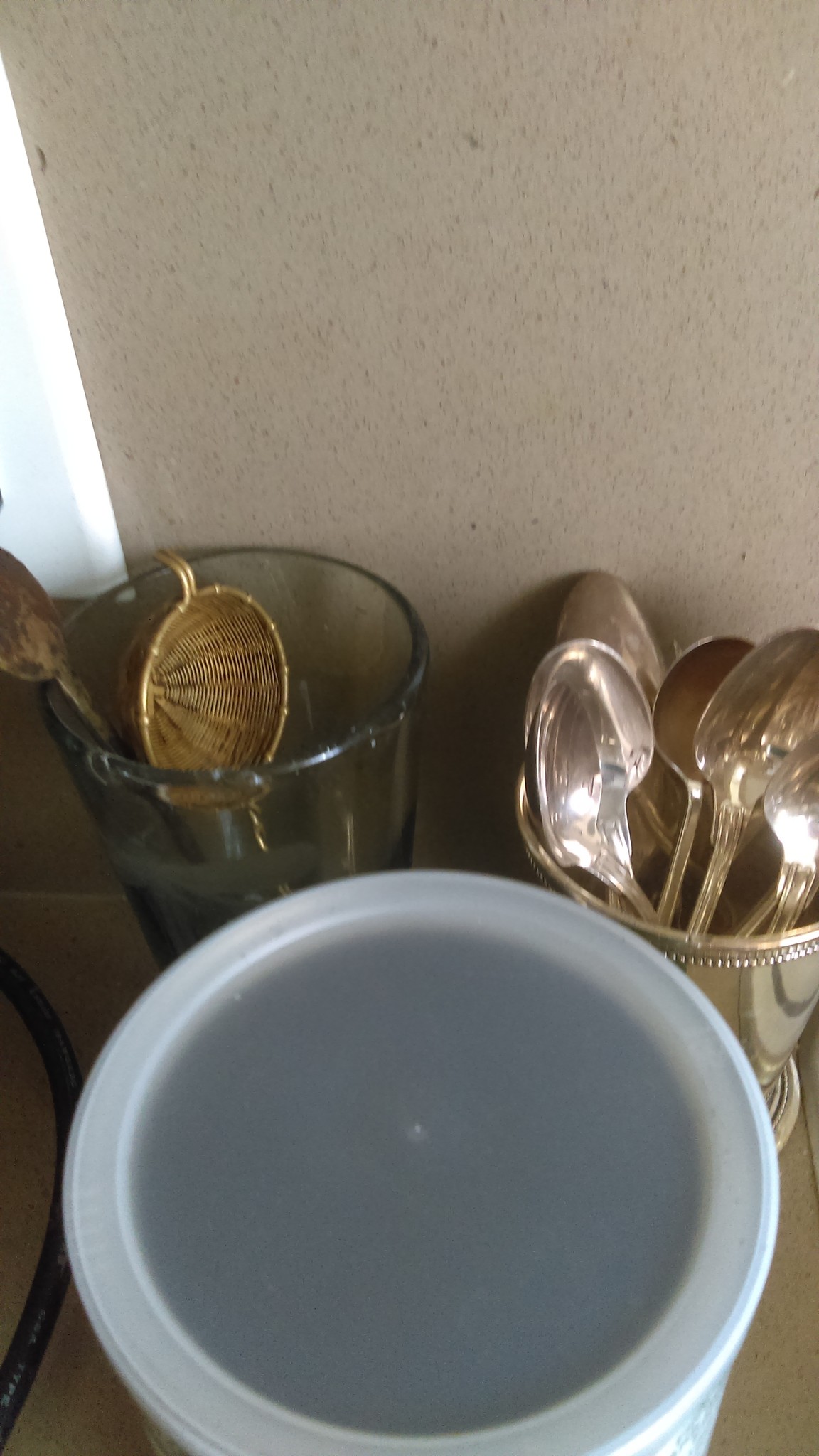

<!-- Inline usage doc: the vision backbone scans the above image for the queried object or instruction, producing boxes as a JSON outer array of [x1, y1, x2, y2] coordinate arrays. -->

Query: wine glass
[[510, 740, 818, 1153]]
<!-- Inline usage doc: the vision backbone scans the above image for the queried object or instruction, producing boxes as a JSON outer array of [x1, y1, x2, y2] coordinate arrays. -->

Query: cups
[[45, 544, 430, 966], [55, 865, 782, 1456]]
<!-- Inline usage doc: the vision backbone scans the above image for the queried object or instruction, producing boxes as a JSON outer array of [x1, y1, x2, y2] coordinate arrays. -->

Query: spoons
[[520, 571, 818, 943]]
